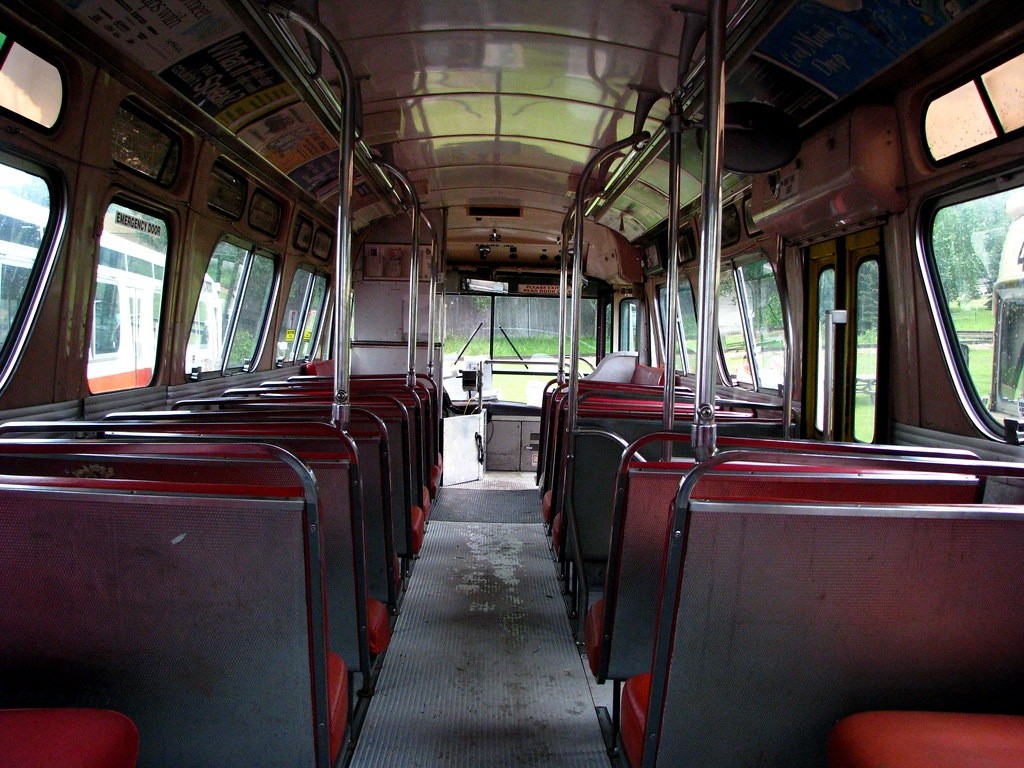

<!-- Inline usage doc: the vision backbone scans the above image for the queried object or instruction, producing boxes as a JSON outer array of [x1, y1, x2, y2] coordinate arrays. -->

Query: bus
[[0, 190, 227, 409], [987, 190, 1024, 429]]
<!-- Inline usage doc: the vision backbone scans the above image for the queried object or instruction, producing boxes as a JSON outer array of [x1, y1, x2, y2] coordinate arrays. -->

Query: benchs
[[0, 364, 1024, 768]]
